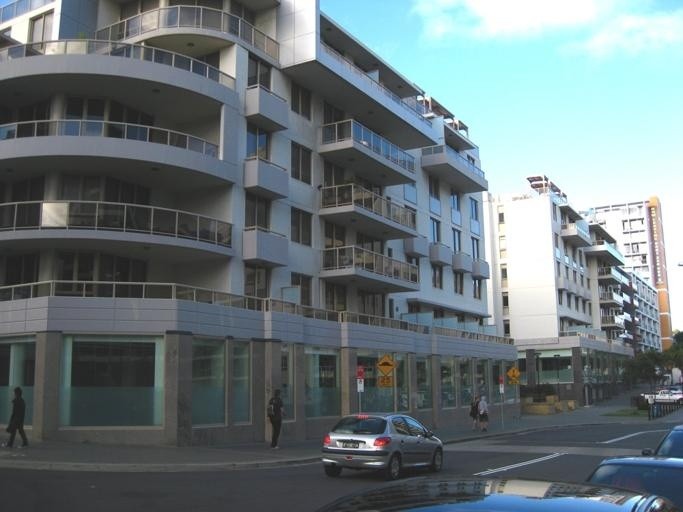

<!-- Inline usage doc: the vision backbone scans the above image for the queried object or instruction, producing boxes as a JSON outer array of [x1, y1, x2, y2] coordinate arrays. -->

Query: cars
[[641, 425, 681, 458], [653, 364, 682, 390], [585, 456, 682, 512], [320, 412, 445, 481], [313, 472, 677, 512], [394, 383, 496, 411]]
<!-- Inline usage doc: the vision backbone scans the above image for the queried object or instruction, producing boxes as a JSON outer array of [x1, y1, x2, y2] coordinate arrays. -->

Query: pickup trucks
[[641, 390, 682, 407]]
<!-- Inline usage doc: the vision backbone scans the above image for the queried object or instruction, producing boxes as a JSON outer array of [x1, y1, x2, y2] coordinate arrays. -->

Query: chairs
[[611, 474, 648, 494]]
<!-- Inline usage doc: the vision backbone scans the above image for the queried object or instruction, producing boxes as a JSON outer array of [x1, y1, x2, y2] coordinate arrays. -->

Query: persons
[[477, 395, 488, 432], [469, 396, 480, 431], [5, 386, 29, 448], [266, 389, 286, 449]]
